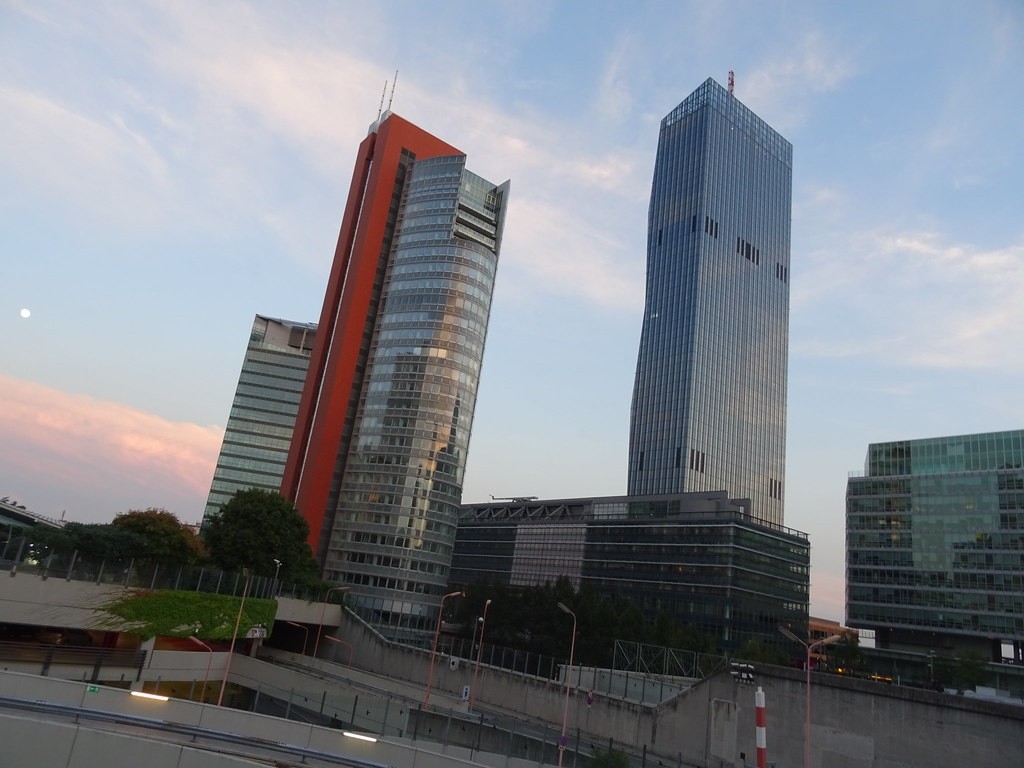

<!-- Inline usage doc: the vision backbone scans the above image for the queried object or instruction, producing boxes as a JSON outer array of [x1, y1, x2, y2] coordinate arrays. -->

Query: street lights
[[325, 636, 353, 667], [189, 635, 213, 703], [312, 586, 350, 658], [467, 599, 492, 714], [217, 568, 249, 706], [287, 621, 309, 654], [424, 591, 462, 710], [556, 603, 577, 768], [263, 560, 283, 627], [777, 626, 841, 768]]
